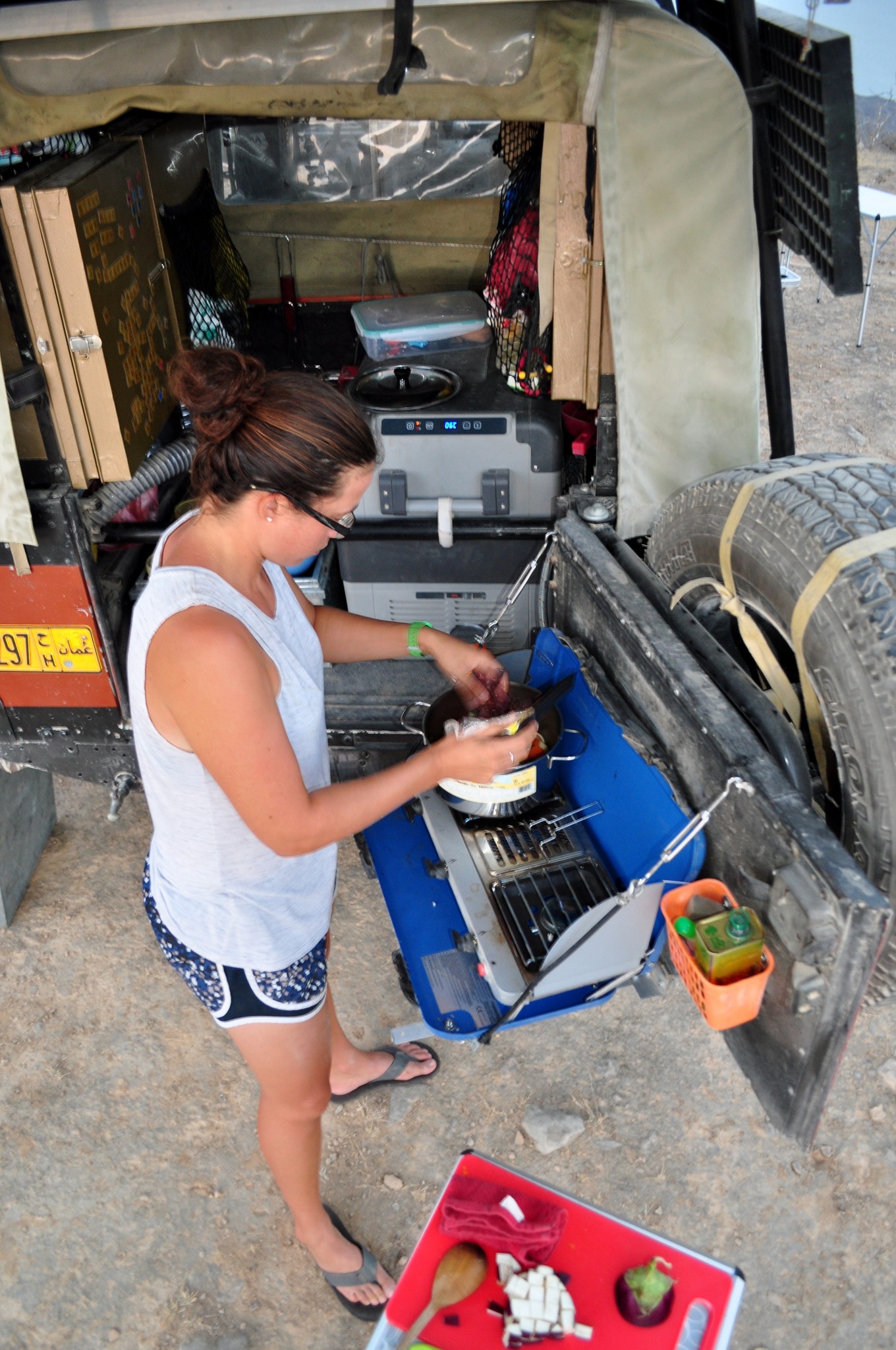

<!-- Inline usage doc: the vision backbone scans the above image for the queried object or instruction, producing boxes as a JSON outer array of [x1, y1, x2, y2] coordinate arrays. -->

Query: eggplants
[[613, 1256, 674, 1328]]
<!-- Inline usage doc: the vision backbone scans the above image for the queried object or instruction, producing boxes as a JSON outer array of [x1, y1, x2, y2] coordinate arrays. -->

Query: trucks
[[0, 0, 866, 825]]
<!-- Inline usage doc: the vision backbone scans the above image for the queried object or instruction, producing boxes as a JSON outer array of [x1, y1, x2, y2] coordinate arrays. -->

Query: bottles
[[675, 915, 695, 950], [695, 904, 766, 986]]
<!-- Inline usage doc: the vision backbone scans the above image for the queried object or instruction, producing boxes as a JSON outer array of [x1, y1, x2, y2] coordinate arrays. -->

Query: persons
[[127, 344, 541, 1320]]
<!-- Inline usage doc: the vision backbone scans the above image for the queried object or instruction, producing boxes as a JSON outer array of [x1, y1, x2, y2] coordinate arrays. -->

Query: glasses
[[252, 485, 357, 542]]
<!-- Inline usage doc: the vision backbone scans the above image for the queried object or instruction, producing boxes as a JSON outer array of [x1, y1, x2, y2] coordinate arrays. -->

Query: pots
[[400, 680, 587, 817]]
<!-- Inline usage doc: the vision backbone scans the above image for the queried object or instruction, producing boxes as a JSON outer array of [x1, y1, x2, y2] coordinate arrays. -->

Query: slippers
[[328, 1041, 440, 1104], [295, 1202, 398, 1321]]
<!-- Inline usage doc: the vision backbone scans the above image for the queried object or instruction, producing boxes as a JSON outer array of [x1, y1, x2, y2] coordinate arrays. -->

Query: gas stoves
[[410, 739, 622, 1006]]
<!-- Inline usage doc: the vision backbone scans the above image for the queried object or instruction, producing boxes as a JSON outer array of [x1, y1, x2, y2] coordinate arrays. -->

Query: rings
[[510, 751, 515, 764]]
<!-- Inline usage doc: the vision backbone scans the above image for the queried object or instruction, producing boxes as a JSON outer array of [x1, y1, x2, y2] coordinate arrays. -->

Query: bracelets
[[407, 621, 433, 659]]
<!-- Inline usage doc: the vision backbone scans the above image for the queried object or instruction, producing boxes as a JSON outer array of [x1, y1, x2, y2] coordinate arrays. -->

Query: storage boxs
[[349, 291, 496, 388]]
